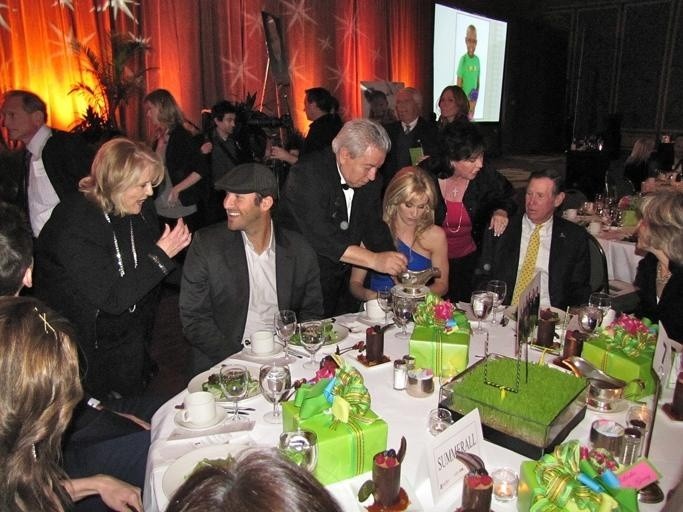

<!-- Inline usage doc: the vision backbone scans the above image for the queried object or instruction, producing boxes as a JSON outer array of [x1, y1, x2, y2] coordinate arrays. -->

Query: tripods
[[265, 130, 287, 204]]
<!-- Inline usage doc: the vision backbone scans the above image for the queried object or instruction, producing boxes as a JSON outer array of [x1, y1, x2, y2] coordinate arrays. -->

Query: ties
[[510, 222, 544, 308], [22, 153, 34, 237], [402, 125, 412, 137]]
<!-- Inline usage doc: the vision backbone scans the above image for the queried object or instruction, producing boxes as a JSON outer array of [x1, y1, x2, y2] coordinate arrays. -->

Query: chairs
[[562, 189, 588, 210], [614, 175, 635, 195]]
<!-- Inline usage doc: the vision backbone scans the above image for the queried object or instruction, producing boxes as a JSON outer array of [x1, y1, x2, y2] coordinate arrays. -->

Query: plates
[[173, 407, 226, 431], [241, 343, 283, 356], [284, 321, 349, 348], [188, 363, 270, 402], [163, 442, 266, 504]]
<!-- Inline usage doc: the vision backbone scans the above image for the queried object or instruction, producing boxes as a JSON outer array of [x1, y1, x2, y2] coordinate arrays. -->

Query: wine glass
[[274, 309, 324, 371], [219, 363, 293, 427]]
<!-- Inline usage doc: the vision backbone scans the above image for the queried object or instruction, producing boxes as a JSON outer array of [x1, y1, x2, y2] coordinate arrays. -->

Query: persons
[[459, 25, 481, 119], [0, 85, 683, 512]]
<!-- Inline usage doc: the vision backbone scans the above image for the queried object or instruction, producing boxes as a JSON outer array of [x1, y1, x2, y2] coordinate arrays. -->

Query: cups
[[363, 266, 653, 512], [242, 332, 275, 352], [278, 430, 317, 473], [179, 392, 216, 424], [563, 194, 622, 236]]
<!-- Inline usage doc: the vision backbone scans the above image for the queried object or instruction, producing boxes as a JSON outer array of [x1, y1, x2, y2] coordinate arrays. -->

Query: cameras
[[247, 114, 294, 130]]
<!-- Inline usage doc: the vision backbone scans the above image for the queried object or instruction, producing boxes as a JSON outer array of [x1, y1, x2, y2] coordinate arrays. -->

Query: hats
[[214, 162, 278, 202]]
